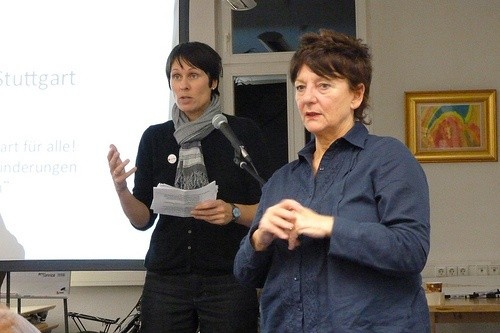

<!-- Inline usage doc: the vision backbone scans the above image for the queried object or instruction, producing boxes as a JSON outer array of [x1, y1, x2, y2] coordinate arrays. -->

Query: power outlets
[[435, 267, 468, 277]]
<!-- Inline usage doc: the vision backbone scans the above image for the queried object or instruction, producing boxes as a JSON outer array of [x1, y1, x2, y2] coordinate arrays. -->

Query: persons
[[106, 41, 257, 333], [234, 27, 433, 333]]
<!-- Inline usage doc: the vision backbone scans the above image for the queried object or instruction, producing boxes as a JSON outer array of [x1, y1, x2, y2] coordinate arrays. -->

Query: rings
[[114, 170, 117, 177], [289, 227, 294, 232]]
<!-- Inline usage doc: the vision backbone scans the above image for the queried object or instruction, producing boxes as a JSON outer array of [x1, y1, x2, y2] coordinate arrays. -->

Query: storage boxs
[[425, 292, 444, 306]]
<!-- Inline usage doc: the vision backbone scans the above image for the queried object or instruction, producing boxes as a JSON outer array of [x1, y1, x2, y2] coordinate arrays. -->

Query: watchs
[[229, 202, 240, 225]]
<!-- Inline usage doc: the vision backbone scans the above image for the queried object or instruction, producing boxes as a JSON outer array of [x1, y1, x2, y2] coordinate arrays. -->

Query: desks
[[428, 297, 500, 333], [11, 304, 60, 333]]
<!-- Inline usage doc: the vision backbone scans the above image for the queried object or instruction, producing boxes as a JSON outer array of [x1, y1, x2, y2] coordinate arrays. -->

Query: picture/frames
[[403, 89, 499, 162]]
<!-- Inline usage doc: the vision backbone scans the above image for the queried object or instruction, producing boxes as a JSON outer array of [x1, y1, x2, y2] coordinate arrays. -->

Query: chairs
[[66, 293, 143, 333]]
